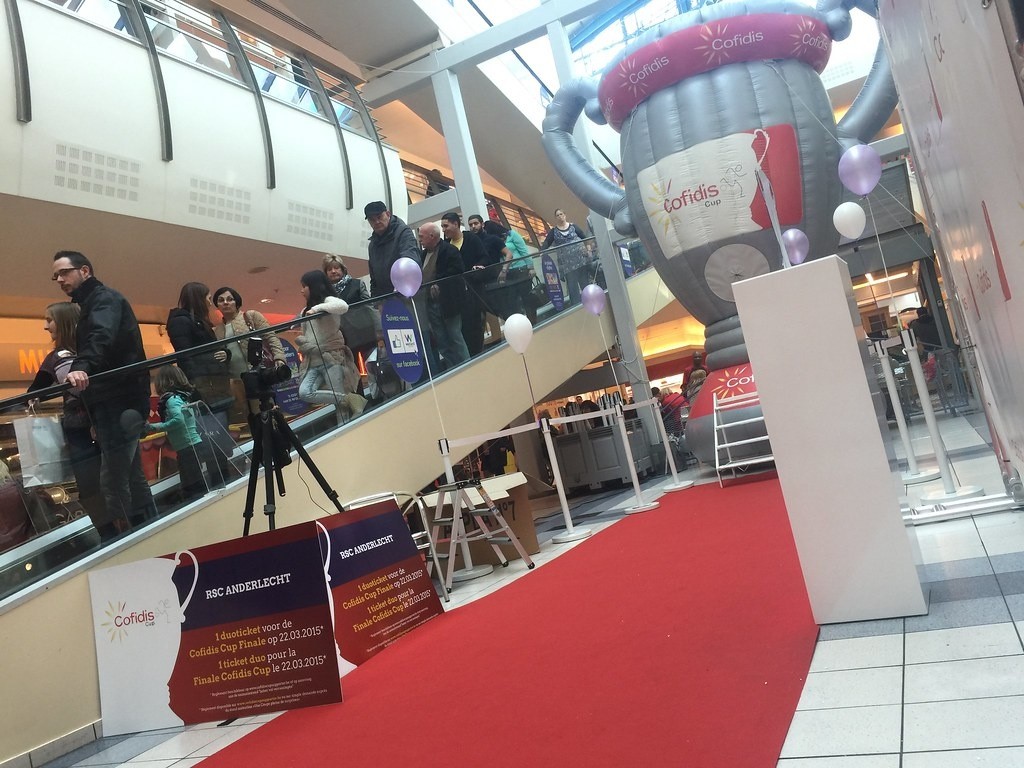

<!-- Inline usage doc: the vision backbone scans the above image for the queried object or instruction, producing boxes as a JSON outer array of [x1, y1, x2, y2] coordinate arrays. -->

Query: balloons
[[581, 284, 607, 317], [780, 226, 811, 266], [505, 313, 533, 355], [836, 142, 883, 196], [391, 255, 423, 297]]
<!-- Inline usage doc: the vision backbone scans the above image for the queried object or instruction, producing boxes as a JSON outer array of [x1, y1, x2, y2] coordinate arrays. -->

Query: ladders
[[426, 477, 535, 592]]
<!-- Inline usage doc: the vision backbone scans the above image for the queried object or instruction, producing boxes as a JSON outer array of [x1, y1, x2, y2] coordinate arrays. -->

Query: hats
[[364, 201, 387, 220]]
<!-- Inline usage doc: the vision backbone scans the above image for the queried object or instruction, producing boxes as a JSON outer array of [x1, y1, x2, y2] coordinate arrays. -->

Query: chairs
[[339, 490, 450, 602], [874, 348, 968, 430]]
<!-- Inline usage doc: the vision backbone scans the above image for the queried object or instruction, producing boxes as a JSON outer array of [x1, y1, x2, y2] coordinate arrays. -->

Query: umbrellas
[[831, 202, 867, 242]]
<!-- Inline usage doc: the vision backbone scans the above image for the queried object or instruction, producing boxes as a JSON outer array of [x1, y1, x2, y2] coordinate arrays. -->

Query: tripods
[[243, 397, 344, 538]]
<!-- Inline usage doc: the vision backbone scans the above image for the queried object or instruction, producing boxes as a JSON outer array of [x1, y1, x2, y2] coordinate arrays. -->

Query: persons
[[0, 459, 40, 554], [480, 352, 710, 480], [909, 307, 942, 351], [298, 270, 368, 428], [27, 301, 133, 549], [50, 250, 159, 535], [145, 365, 210, 502], [364, 201, 433, 404], [324, 253, 377, 415], [166, 282, 232, 489], [415, 168, 610, 371], [213, 287, 286, 466]]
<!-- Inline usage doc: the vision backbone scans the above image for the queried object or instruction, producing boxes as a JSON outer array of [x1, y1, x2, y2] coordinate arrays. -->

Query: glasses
[[217, 298, 235, 303], [51, 267, 81, 281]]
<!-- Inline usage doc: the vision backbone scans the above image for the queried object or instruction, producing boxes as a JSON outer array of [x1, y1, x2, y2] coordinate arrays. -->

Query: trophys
[[543, 1, 900, 473]]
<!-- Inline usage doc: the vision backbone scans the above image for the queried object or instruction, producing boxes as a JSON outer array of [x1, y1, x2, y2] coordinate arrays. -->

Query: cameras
[[241, 336, 292, 399]]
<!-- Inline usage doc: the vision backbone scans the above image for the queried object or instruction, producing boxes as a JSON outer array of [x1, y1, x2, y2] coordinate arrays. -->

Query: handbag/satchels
[[375, 357, 399, 385], [243, 312, 276, 368], [13, 404, 76, 489], [527, 275, 551, 308]]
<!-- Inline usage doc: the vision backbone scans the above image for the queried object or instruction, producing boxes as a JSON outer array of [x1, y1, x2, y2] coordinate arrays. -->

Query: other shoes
[[344, 392, 368, 419]]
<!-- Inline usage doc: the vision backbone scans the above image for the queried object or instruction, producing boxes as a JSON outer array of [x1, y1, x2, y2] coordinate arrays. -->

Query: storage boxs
[[414, 471, 540, 579]]
